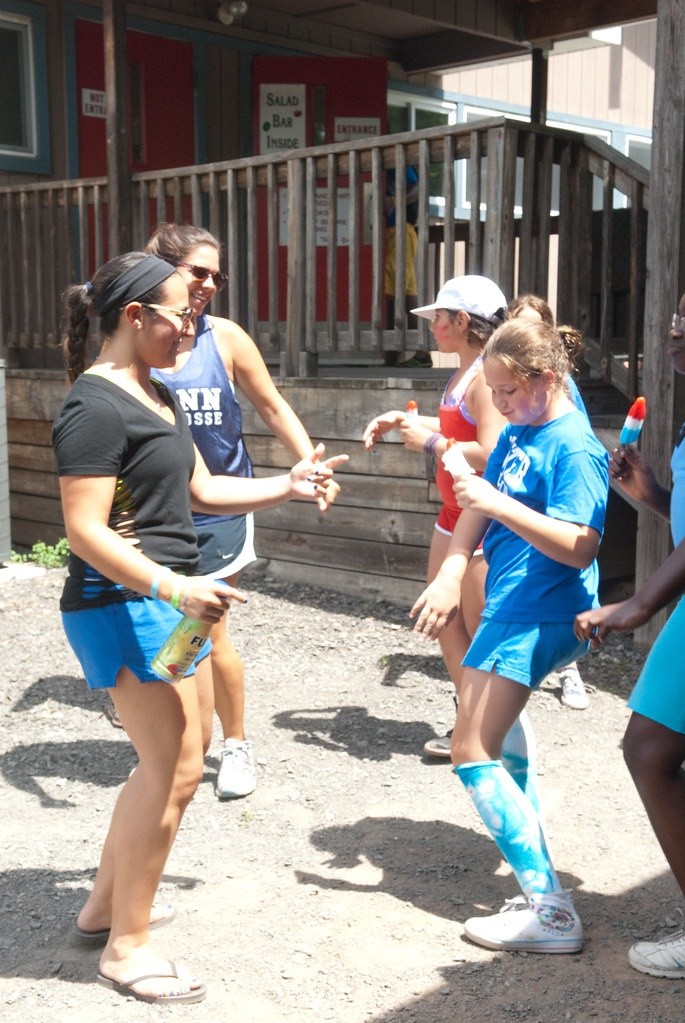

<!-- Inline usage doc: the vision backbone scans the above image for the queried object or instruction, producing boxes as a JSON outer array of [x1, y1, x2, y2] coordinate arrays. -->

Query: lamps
[[219, 1, 248, 27]]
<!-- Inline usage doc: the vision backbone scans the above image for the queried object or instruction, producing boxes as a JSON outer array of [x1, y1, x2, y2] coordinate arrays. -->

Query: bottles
[[150, 578, 232, 684]]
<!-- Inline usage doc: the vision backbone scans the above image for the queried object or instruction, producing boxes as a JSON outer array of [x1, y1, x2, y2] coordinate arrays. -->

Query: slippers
[[97, 959, 208, 1004], [71, 897, 177, 946]]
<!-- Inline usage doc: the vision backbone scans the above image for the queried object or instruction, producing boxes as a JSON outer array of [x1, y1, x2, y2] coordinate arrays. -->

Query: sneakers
[[628, 908, 685, 979], [214, 737, 255, 798], [464, 887, 582, 953], [424, 692, 460, 757], [559, 667, 597, 710]]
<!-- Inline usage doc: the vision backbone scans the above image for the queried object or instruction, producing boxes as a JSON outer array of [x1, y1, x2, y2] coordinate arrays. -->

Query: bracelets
[[179, 576, 193, 611], [171, 574, 185, 609], [425, 432, 444, 455], [150, 567, 170, 600]]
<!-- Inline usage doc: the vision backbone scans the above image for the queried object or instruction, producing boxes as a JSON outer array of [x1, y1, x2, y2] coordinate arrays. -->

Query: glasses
[[671, 313, 685, 331], [171, 260, 229, 292], [118, 302, 194, 330]]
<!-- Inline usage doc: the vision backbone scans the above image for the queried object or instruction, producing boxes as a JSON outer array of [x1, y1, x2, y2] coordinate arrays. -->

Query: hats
[[410, 275, 507, 326]]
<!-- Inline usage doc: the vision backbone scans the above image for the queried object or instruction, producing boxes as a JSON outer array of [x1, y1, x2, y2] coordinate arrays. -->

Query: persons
[[385, 167, 433, 369], [409, 318, 610, 954], [363, 275, 509, 756], [572, 426, 685, 979], [50, 251, 349, 1002], [145, 223, 340, 798]]
